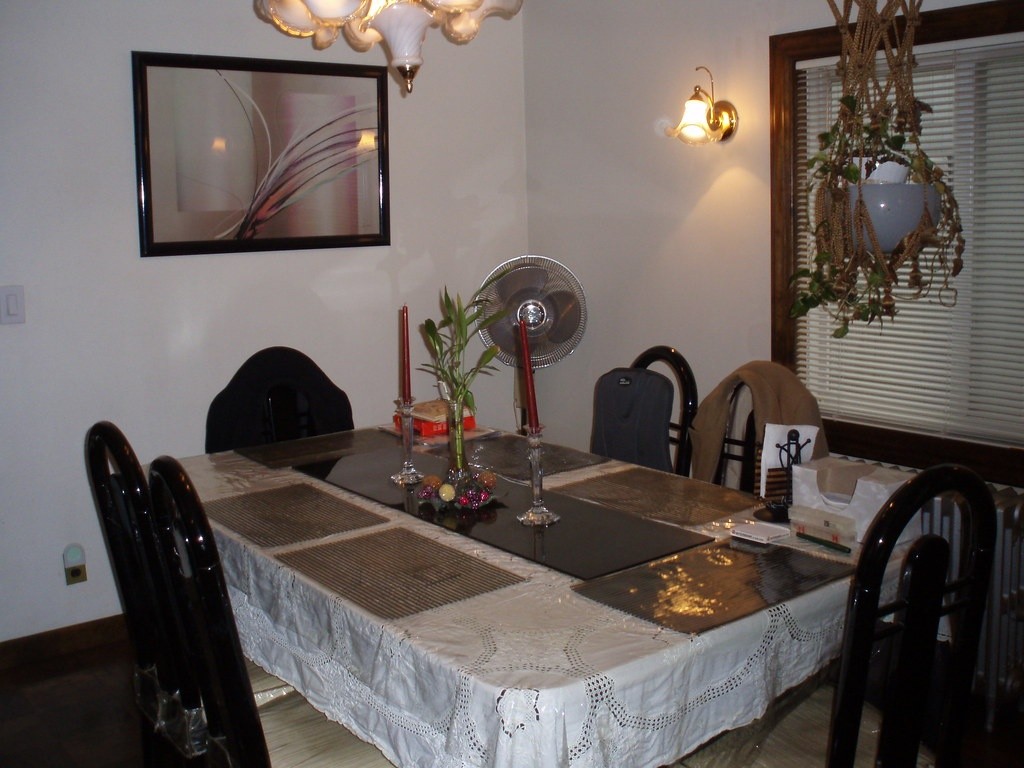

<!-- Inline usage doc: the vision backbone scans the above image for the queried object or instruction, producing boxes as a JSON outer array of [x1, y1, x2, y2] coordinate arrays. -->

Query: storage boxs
[[791, 456, 922, 548], [393, 415, 476, 439]]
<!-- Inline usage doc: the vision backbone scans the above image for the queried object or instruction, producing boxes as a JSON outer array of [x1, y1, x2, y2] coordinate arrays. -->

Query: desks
[[133, 420, 903, 768]]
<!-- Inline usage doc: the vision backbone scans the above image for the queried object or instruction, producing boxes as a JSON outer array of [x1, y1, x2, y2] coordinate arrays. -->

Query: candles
[[401, 301, 411, 405], [518, 317, 539, 434]]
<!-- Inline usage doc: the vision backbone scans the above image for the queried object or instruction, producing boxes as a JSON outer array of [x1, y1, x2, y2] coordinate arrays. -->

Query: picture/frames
[[130, 50, 390, 258]]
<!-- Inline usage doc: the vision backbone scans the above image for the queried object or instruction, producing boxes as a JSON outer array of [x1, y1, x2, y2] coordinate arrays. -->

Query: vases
[[445, 402, 475, 484]]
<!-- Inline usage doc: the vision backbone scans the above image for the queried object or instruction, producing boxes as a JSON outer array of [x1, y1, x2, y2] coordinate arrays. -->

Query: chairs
[[84, 346, 395, 768], [824, 462, 1000, 768], [591, 346, 830, 498]]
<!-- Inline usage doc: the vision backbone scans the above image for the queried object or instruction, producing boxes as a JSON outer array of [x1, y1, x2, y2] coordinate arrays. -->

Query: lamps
[[252, 0, 526, 94], [664, 66, 739, 148]]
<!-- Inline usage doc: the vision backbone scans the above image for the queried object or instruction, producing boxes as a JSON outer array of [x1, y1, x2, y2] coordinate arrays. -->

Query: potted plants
[[781, 93, 962, 338]]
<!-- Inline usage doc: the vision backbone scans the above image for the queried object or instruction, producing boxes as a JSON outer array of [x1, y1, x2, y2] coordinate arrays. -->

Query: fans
[[473, 254, 588, 437]]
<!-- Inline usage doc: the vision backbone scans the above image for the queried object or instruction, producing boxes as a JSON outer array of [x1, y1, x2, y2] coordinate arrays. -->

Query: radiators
[[828, 452, 1024, 735]]
